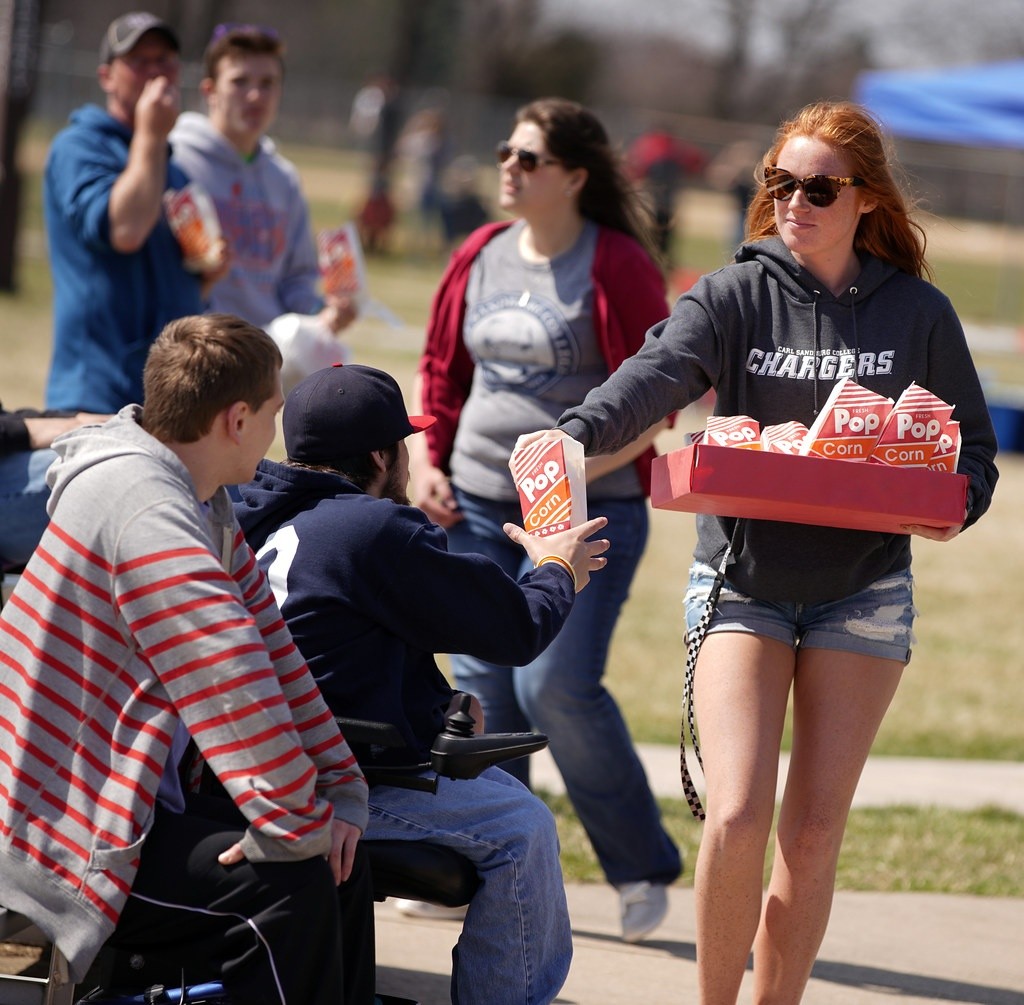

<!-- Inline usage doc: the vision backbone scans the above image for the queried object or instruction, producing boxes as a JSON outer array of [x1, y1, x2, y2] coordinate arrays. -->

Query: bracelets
[[535, 554, 577, 592]]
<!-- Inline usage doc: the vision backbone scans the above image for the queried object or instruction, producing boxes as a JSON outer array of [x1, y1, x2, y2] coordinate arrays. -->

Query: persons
[[0, 313, 378, 1005], [350, 71, 705, 270], [406, 91, 684, 947], [0, 0, 370, 587], [508, 100, 1000, 1005], [230, 363, 612, 1005]]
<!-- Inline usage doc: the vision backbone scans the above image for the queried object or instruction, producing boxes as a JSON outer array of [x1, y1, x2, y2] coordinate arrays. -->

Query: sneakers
[[397, 899, 469, 920], [619, 880, 666, 941]]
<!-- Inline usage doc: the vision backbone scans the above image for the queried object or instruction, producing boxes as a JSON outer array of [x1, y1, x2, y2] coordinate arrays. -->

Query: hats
[[283, 362, 437, 465], [99, 12, 182, 66]]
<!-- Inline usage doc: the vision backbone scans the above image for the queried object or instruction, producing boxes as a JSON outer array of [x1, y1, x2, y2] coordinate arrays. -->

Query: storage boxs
[[319, 224, 365, 306], [651, 376, 967, 535], [168, 184, 219, 264], [514, 435, 588, 537]]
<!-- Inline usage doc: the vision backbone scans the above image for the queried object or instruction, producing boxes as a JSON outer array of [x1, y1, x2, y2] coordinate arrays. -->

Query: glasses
[[497, 142, 567, 173], [764, 166, 865, 207]]
